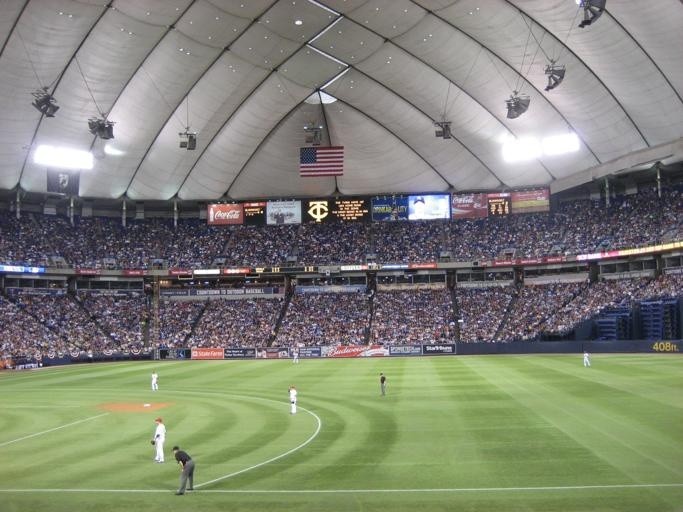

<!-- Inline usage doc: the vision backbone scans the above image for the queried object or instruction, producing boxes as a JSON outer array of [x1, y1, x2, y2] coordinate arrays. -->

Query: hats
[[173, 447, 178, 450], [155, 418, 161, 422]]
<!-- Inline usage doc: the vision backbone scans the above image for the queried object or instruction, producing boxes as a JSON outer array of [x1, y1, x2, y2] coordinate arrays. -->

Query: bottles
[[208, 208, 213, 222]]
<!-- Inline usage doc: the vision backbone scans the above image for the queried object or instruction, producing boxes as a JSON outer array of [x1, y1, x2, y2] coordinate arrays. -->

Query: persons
[[154, 417, 165, 463], [380, 373, 386, 395], [151, 371, 159, 391], [288, 385, 297, 414], [173, 446, 194, 494]]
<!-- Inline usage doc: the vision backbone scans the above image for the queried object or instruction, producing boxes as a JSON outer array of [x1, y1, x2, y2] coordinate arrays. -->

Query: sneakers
[[176, 488, 193, 494], [154, 458, 164, 463]]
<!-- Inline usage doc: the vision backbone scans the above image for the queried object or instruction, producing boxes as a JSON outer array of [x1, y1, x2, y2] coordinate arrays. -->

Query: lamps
[[32, 0, 611, 151]]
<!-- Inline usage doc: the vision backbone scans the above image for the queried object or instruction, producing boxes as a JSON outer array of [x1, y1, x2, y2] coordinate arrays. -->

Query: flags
[[300, 146, 344, 176]]
